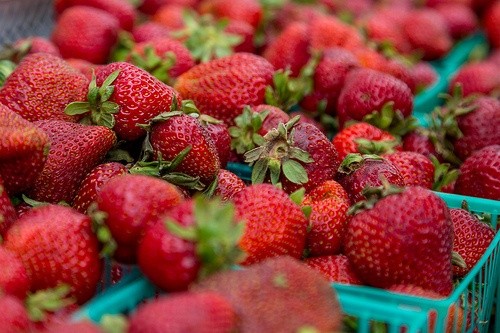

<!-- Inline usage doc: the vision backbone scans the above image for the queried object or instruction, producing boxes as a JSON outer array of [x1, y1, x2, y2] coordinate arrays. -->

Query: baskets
[[232, 181, 500, 333], [69, 267, 428, 333]]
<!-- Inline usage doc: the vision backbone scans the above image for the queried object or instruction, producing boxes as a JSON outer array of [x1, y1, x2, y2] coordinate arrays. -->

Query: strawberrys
[[0, 0, 500, 333]]
